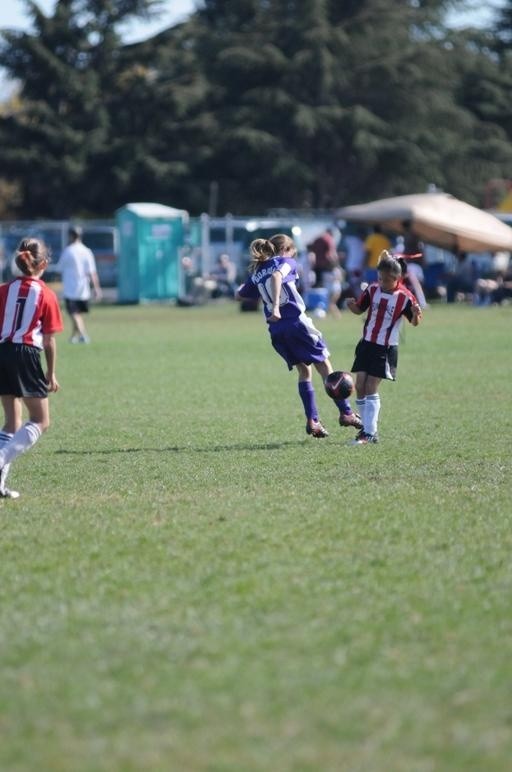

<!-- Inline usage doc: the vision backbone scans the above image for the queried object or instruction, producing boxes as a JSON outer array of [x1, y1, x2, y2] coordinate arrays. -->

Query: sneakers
[[353, 429, 378, 445], [307, 418, 329, 437], [70, 336, 89, 345], [340, 413, 362, 429], [1, 488, 20, 499]]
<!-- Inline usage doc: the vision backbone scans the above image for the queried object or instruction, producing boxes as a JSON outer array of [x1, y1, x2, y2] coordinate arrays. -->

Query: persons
[[234, 233, 422, 445], [181, 217, 511, 313], [0, 224, 102, 499]]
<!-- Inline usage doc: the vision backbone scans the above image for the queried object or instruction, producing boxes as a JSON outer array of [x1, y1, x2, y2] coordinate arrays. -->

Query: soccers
[[325, 372, 353, 399], [315, 308, 326, 318]]
